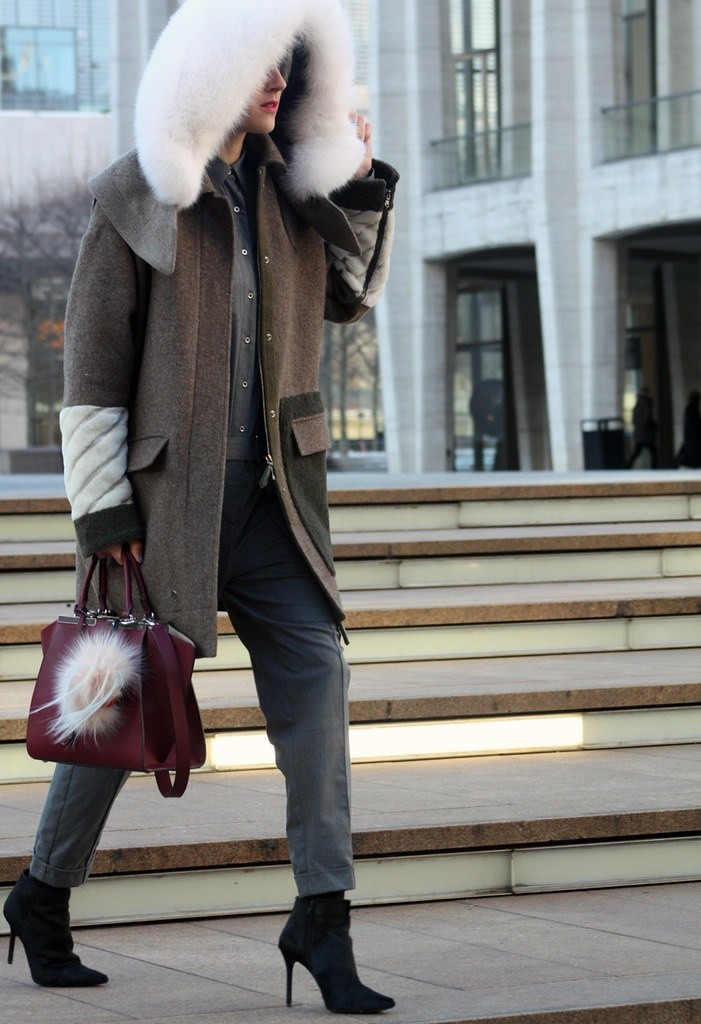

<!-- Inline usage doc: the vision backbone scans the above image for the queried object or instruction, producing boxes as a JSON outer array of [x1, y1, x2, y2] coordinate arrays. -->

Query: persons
[[627, 387, 659, 469], [4, 1, 400, 1015]]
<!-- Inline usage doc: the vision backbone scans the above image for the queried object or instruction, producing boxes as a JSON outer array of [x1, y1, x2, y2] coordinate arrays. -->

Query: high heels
[[3, 868, 108, 987], [278, 897, 395, 1014]]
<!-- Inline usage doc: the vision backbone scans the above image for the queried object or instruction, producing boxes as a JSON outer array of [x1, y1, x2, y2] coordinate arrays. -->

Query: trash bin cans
[[580, 417, 625, 471]]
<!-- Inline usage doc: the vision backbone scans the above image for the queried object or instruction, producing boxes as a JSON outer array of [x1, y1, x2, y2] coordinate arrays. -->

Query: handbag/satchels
[[25, 545, 206, 799]]
